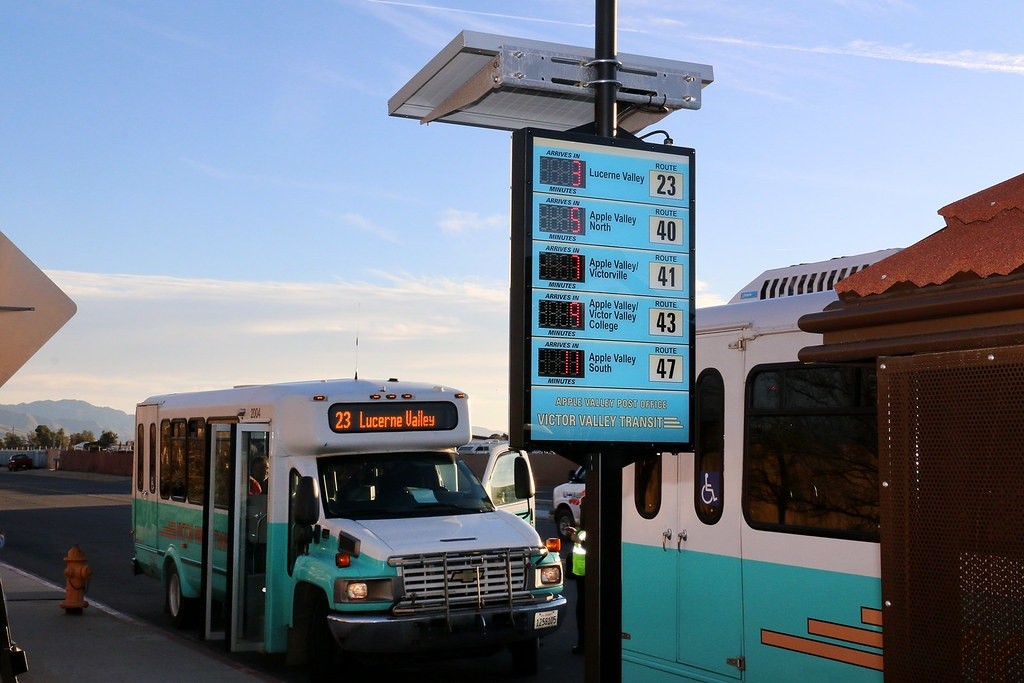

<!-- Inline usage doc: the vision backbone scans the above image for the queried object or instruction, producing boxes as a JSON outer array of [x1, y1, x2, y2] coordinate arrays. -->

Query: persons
[[562, 526, 586, 654], [250, 455, 269, 495]]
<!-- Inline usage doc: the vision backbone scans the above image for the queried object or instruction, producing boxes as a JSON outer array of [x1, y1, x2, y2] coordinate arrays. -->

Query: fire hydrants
[[59, 543, 92, 615]]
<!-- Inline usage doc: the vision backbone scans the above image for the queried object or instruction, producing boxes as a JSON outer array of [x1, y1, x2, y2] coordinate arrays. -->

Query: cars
[[8, 454, 33, 472], [548, 465, 586, 540]]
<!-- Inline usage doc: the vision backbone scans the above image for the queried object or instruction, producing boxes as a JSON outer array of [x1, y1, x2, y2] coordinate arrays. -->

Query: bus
[[128, 379, 565, 683], [621, 248, 905, 683], [74, 442, 102, 451]]
